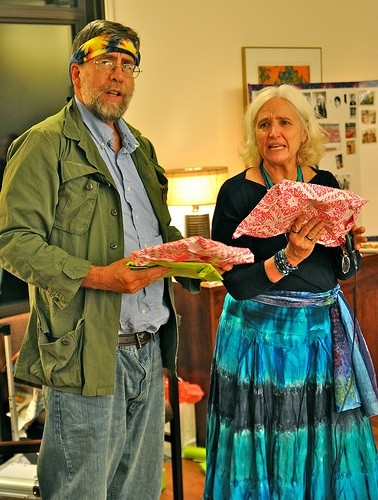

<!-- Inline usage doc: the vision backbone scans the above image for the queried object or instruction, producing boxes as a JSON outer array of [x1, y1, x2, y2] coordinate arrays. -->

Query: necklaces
[[259, 158, 307, 243]]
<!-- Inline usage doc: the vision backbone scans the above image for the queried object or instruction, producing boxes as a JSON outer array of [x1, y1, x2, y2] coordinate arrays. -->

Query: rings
[[306, 235, 312, 241], [290, 228, 298, 233]]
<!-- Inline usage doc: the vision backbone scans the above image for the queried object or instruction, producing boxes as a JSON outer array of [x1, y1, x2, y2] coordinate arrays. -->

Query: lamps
[[163, 166, 229, 239]]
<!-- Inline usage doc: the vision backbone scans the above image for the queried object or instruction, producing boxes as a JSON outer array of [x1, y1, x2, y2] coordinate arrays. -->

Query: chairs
[[0, 300, 185, 500]]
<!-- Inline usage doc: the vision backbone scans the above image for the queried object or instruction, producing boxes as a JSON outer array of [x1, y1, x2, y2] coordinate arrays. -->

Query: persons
[[211, 78, 378, 500], [0, 19, 233, 500], [333, 97, 340, 108], [316, 93, 328, 118], [350, 94, 355, 105]]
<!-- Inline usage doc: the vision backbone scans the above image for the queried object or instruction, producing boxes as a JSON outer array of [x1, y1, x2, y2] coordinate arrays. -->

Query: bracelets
[[273, 249, 298, 276]]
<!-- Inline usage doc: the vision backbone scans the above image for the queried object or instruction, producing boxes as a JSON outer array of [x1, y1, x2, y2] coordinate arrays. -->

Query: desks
[[172, 239, 378, 449]]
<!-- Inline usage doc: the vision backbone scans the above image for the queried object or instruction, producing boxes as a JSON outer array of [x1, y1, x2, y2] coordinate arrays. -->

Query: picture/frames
[[241, 46, 323, 115]]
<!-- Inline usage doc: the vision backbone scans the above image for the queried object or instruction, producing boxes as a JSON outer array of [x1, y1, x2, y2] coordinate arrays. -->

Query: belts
[[118, 327, 161, 348]]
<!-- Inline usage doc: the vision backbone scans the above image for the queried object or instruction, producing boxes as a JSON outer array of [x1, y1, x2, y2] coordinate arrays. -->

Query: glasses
[[85, 59, 142, 79]]
